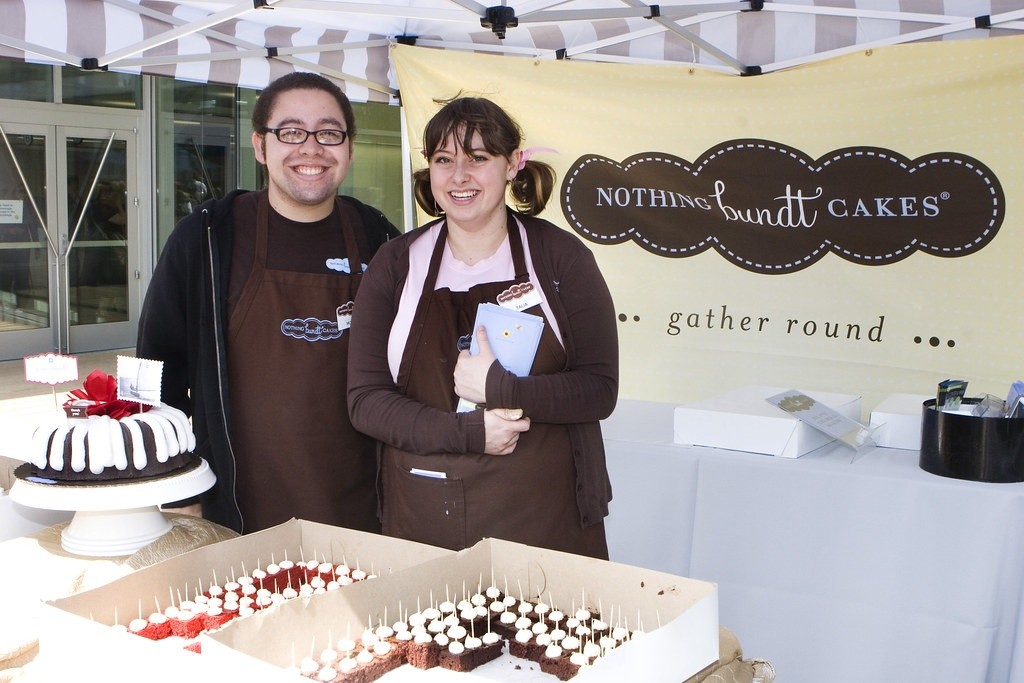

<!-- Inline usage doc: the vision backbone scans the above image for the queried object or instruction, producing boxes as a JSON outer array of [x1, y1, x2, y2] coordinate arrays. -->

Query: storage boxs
[[1004, 379, 1024, 418], [42, 517, 456, 683], [672, 394, 860, 460], [867, 393, 932, 451], [973, 393, 1008, 417], [204, 538, 719, 683]]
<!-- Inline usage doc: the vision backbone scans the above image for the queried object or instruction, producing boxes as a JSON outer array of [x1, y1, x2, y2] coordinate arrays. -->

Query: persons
[[67, 156, 126, 286], [2, 183, 41, 308], [137, 69, 404, 538], [347, 98, 619, 562]]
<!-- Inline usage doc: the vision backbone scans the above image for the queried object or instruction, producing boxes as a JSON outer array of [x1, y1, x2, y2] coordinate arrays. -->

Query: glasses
[[261, 128, 350, 146]]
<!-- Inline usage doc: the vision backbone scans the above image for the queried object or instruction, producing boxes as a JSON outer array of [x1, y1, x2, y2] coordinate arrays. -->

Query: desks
[[600, 398, 1024, 683]]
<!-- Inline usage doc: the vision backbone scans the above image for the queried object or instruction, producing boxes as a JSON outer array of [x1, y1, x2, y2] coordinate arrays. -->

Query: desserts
[[109, 545, 633, 683]]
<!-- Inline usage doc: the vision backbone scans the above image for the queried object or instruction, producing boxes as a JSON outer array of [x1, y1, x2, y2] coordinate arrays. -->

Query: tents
[[2, 1, 1024, 231]]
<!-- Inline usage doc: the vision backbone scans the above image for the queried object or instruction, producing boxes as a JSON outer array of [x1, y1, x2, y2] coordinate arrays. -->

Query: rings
[[505, 408, 509, 420]]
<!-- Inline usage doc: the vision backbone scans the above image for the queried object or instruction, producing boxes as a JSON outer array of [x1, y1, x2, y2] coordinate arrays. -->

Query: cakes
[[30, 398, 198, 480]]
